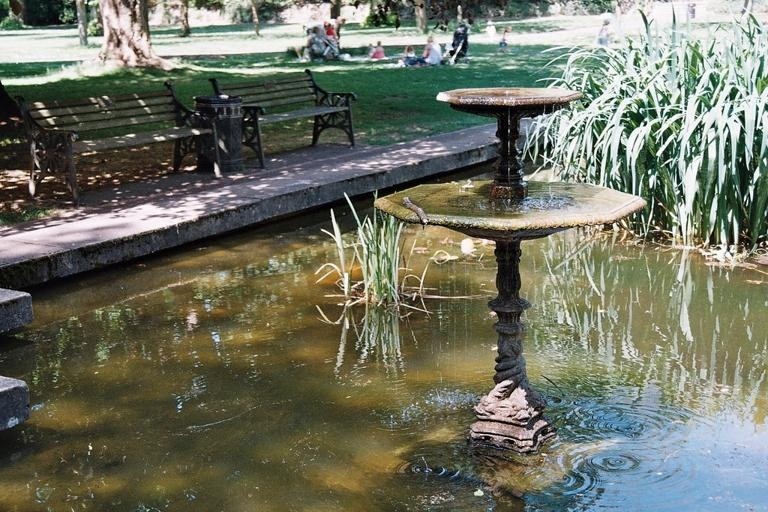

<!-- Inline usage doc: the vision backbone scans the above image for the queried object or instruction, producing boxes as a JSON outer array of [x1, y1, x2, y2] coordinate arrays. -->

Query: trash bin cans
[[192, 95, 243, 173]]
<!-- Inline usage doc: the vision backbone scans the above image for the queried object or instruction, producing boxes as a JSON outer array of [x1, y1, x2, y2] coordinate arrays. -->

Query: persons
[[597, 20, 609, 46], [290, 3, 514, 68]]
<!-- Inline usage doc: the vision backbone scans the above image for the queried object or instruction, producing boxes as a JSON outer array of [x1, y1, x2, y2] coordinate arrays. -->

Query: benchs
[[208, 69, 357, 169], [15, 80, 221, 208]]
